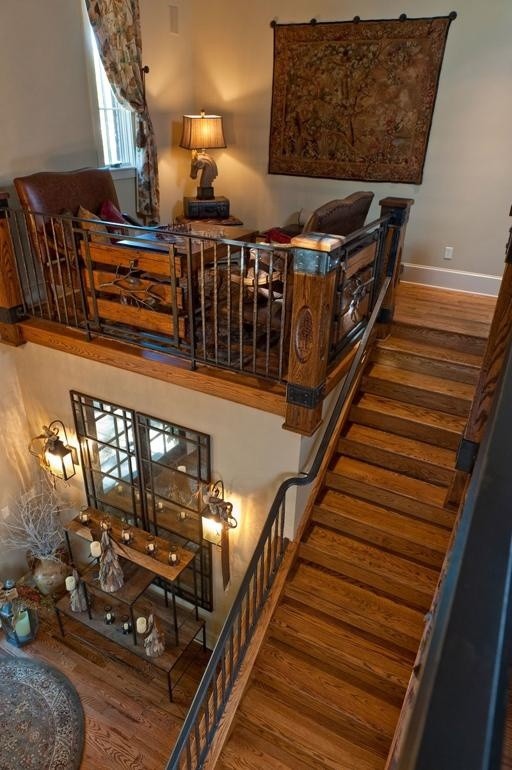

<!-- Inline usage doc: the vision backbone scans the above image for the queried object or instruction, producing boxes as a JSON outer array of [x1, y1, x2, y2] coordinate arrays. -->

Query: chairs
[[13, 166, 146, 326], [199, 190, 375, 366]]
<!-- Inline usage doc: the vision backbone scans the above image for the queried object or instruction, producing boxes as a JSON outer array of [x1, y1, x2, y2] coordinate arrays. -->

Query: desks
[[62, 507, 199, 647], [115, 222, 259, 320], [78, 556, 168, 646], [53, 582, 207, 703]]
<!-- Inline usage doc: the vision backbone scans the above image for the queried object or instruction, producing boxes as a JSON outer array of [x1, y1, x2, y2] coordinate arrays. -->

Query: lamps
[[178, 108, 230, 220], [201, 480, 238, 547], [28, 420, 79, 481]]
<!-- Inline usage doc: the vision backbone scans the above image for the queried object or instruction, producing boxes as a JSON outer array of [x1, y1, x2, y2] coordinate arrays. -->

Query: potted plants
[[0, 476, 72, 597]]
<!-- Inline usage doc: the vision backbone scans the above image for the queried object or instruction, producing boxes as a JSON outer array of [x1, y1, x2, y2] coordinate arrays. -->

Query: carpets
[[0, 640, 86, 770]]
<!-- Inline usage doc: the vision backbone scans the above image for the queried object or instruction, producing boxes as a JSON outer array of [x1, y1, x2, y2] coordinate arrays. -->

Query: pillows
[[50, 208, 79, 267], [101, 201, 136, 243], [78, 204, 111, 266]]
